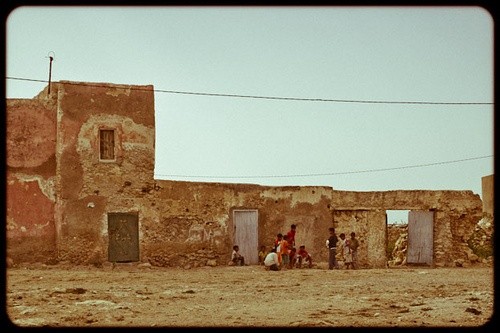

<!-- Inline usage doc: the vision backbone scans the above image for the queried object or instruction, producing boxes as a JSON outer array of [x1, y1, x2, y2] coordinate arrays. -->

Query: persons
[[273, 233, 284, 266], [286, 224, 296, 249], [265, 249, 280, 271], [231, 245, 247, 266], [326, 227, 339, 270], [297, 244, 314, 269], [348, 231, 360, 270], [282, 234, 295, 269], [258, 245, 269, 265], [339, 232, 350, 270]]
